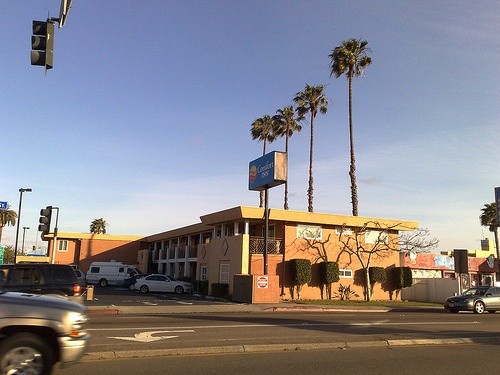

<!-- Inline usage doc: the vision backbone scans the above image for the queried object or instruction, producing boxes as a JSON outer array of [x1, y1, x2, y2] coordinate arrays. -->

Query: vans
[[85, 262, 141, 287], [0, 261, 86, 375]]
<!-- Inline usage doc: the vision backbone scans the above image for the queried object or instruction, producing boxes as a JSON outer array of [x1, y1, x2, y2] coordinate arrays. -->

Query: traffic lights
[[38, 206, 52, 235], [31, 19, 54, 76]]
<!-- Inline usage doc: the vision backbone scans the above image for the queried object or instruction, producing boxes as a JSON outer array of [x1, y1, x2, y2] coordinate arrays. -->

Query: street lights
[[14, 189, 32, 264], [22, 226, 30, 255]]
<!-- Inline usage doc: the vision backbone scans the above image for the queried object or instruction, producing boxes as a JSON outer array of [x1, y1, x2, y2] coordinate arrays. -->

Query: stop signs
[[257, 277, 269, 289]]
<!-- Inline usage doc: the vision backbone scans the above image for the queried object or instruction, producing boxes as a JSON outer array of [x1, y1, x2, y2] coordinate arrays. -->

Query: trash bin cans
[[87, 285, 94, 300]]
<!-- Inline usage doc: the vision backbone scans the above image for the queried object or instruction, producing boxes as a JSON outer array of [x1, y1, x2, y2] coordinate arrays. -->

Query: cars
[[444, 286, 500, 313], [123, 274, 193, 295]]
